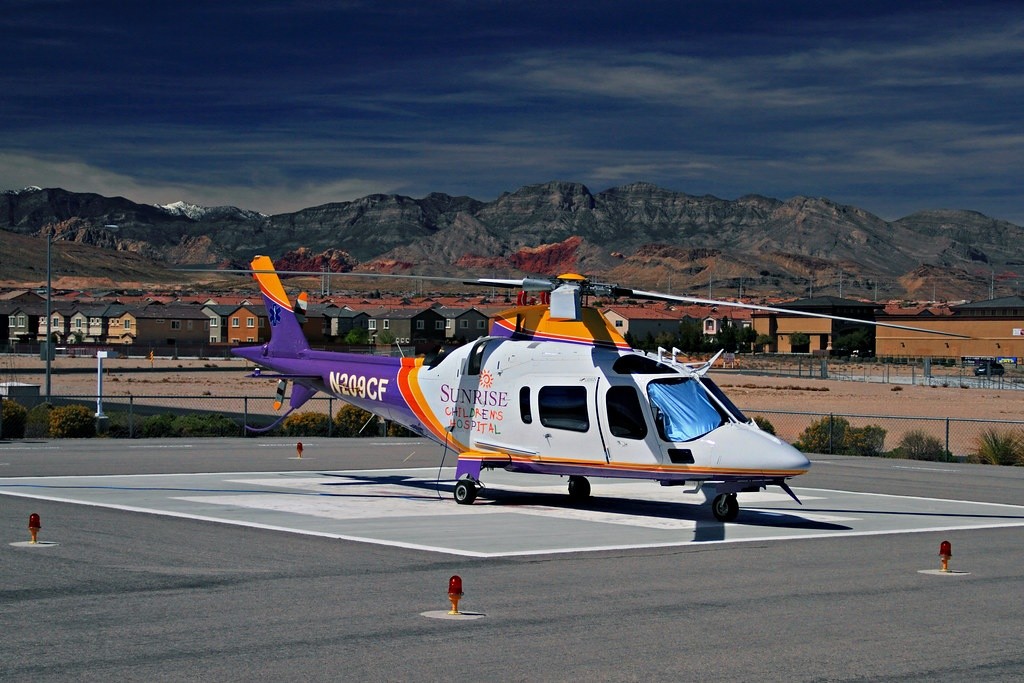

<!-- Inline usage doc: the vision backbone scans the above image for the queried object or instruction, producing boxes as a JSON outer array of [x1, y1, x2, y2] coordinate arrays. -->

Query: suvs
[[974, 362, 1004, 376]]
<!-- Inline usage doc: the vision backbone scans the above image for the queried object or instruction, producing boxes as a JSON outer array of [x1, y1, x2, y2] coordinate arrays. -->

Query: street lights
[[46, 224, 120, 401]]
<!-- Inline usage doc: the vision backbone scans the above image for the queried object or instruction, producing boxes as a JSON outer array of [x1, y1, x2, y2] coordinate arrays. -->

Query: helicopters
[[167, 255, 973, 521]]
